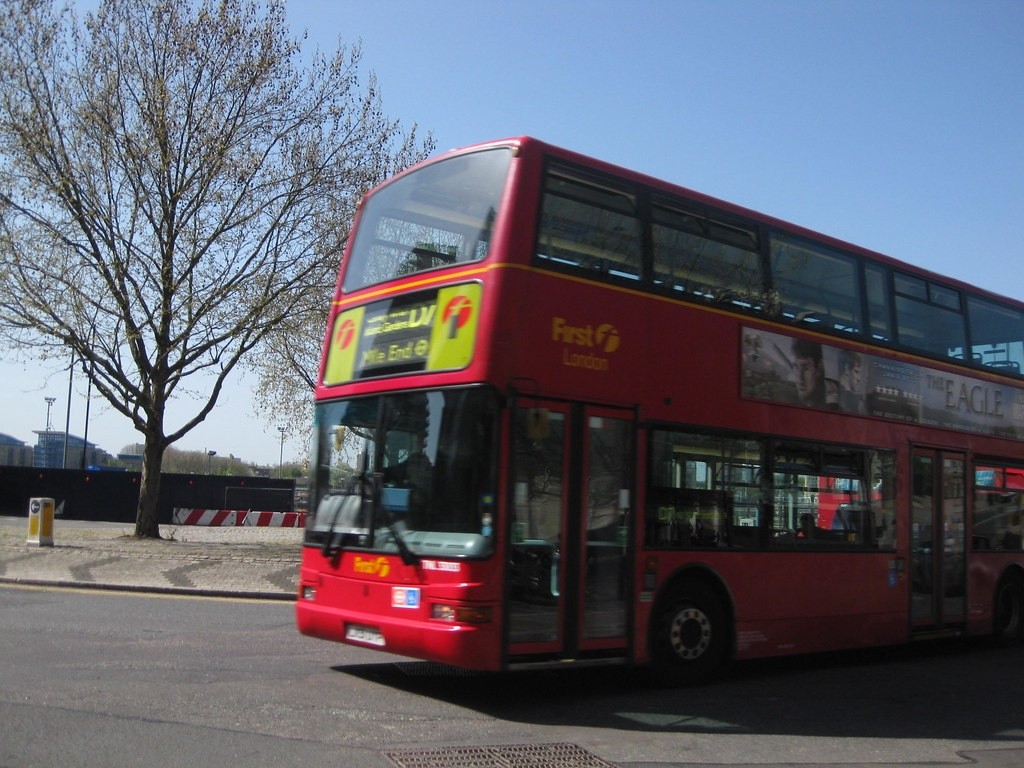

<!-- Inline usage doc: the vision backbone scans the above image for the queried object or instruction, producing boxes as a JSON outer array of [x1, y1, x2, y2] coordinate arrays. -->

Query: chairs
[[950, 350, 1021, 372]]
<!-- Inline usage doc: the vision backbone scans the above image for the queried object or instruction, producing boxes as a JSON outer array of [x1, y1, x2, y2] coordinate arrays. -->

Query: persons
[[798, 514, 822, 538], [366, 421, 428, 487], [791, 341, 845, 412], [832, 348, 869, 414]]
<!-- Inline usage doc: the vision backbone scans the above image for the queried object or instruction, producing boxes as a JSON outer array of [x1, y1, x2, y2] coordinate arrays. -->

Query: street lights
[[278, 426, 287, 478], [43, 397, 55, 468]]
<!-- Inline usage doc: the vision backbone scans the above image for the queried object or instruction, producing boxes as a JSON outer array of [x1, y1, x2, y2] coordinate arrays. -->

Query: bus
[[296, 139, 1024, 692], [721, 464, 1024, 549]]
